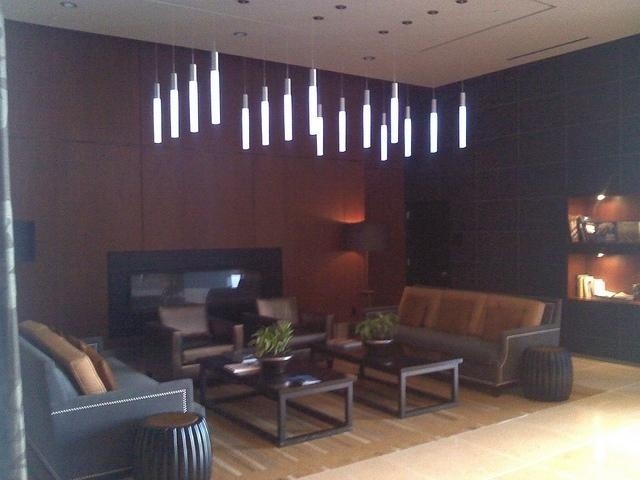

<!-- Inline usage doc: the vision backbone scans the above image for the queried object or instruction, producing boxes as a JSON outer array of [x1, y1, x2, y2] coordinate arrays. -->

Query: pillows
[[66, 334, 120, 390], [396, 285, 546, 343]]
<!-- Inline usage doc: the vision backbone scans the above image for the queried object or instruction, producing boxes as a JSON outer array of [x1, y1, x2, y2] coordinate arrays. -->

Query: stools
[[521, 345, 573, 399], [131, 410, 210, 480]]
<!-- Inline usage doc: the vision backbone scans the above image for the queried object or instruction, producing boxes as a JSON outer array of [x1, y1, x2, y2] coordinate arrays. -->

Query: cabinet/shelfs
[[563, 193, 639, 306]]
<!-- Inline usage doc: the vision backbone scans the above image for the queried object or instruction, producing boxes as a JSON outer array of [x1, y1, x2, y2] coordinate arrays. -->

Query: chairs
[[143, 296, 335, 388]]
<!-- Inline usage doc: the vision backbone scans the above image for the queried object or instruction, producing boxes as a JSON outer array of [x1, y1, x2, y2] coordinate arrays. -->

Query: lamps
[[210, 27, 220, 126], [314, 16, 324, 157], [337, 4, 347, 152], [427, 10, 438, 153], [402, 20, 412, 157], [260, 26, 270, 146], [363, 56, 376, 148], [153, 28, 161, 145], [390, 26, 399, 144], [283, 27, 292, 142], [378, 30, 389, 161], [170, 27, 180, 139], [238, 0, 250, 151], [188, 26, 200, 133], [309, 26, 318, 135], [456, 0, 467, 149]]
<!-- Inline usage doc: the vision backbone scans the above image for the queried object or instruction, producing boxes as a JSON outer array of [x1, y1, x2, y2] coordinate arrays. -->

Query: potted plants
[[250, 319, 298, 375], [354, 312, 400, 346]]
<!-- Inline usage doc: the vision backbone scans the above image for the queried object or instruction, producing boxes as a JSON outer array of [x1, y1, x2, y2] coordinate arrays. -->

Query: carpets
[[303, 383, 640, 479]]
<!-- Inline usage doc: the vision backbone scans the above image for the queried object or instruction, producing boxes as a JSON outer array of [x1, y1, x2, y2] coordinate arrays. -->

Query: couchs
[[15, 317, 200, 479], [357, 279, 563, 396]]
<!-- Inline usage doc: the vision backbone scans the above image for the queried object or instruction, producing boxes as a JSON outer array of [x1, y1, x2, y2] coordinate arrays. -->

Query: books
[[576, 274, 634, 300], [569, 214, 620, 241], [221, 347, 258, 364], [222, 362, 264, 374], [324, 336, 363, 351]]
